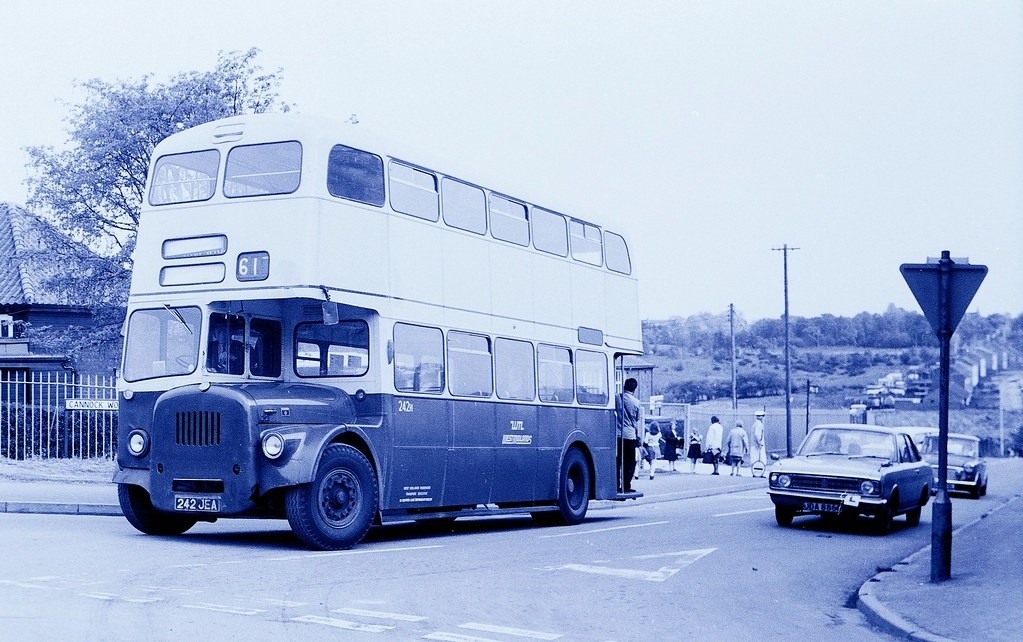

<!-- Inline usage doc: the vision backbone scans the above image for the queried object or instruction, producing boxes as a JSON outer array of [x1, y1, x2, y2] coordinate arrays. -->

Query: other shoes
[[753, 474, 767, 479], [649, 475, 654, 480], [618, 487, 636, 493], [635, 474, 638, 479], [730, 472, 741, 477], [711, 472, 720, 477]]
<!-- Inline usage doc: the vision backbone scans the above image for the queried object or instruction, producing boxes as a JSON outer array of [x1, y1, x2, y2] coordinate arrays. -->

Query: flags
[[809, 386, 818, 394]]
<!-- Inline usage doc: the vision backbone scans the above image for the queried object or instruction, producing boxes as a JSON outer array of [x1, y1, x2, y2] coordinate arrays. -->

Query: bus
[[107, 113, 645, 551]]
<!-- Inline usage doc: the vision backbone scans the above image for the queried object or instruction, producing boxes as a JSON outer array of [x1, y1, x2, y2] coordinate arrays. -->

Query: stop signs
[[752, 461, 766, 477]]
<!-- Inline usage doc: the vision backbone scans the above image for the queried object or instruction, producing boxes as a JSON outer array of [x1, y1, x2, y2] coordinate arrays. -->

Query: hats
[[753, 411, 766, 416]]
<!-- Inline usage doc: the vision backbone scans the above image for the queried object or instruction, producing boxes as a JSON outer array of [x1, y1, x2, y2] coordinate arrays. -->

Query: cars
[[766, 423, 932, 535], [917, 431, 988, 499]]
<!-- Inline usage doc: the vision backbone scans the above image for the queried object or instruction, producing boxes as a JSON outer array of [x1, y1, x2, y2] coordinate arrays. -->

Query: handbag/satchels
[[703, 449, 714, 465], [635, 432, 641, 448]]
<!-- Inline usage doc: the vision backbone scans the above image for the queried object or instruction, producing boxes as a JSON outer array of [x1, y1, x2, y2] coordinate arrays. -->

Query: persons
[[635, 430, 642, 479], [207, 324, 246, 374], [846, 443, 860, 455], [614, 377, 640, 492], [824, 436, 840, 452], [726, 419, 748, 476], [686, 428, 702, 472], [705, 416, 722, 475], [750, 410, 766, 478], [664, 423, 680, 471], [644, 421, 662, 480]]
